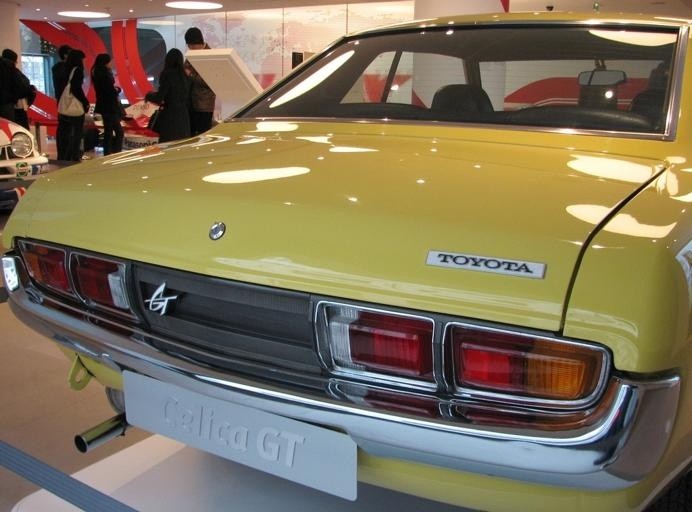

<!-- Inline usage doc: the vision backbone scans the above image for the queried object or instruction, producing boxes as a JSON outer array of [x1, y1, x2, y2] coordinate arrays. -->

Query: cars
[[1, 115, 50, 212], [0, 11, 692, 512]]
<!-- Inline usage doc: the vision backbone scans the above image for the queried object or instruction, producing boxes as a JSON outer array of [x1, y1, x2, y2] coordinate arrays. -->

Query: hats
[[184, 26, 205, 45]]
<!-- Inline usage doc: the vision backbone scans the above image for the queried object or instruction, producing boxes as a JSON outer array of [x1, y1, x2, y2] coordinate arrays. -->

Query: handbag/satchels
[[147, 109, 164, 133], [57, 84, 86, 118]]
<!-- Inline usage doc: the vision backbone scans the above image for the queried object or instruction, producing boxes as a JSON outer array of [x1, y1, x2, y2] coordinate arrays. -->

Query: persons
[[145, 48, 192, 143], [183, 27, 216, 136], [0, 48, 37, 130], [50, 44, 127, 161]]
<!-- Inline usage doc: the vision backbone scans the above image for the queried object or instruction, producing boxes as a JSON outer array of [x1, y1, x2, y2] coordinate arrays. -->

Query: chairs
[[429, 83, 495, 124], [624, 88, 665, 123]]
